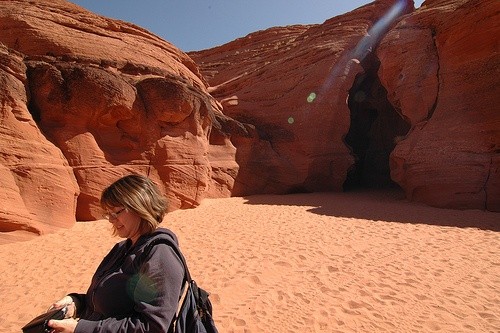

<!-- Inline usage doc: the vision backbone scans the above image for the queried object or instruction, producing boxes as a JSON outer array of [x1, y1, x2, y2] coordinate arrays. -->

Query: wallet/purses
[[21, 304, 69, 333]]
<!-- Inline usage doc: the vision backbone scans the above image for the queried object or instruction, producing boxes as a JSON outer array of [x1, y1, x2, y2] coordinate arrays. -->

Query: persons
[[45, 174, 190, 332]]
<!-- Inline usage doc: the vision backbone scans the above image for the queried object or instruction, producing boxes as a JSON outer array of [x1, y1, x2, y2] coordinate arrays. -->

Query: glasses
[[102, 207, 128, 220]]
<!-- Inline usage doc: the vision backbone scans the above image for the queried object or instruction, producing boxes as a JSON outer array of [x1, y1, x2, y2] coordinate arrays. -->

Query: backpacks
[[126, 234, 219, 333]]
